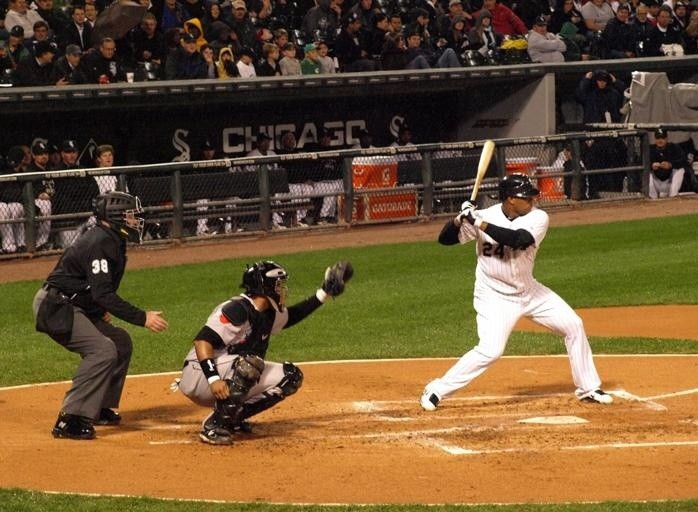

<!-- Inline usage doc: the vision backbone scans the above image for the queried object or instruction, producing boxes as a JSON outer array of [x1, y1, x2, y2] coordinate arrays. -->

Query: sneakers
[[52, 415, 96, 441], [229, 418, 253, 436], [574, 387, 616, 404], [198, 215, 339, 237], [95, 406, 122, 428], [199, 419, 232, 447], [421, 383, 444, 411]]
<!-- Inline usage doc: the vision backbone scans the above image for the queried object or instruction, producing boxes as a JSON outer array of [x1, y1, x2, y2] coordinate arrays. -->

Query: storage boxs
[[350, 154, 399, 193], [335, 186, 419, 228]]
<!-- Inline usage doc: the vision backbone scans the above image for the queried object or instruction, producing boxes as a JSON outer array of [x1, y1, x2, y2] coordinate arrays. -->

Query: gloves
[[456, 199, 484, 232]]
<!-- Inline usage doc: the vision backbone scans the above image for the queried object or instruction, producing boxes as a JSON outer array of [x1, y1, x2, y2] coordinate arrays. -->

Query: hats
[[183, 32, 197, 44], [654, 127, 668, 140], [317, 126, 332, 138], [256, 131, 273, 142], [617, 3, 649, 14], [399, 122, 412, 134], [31, 141, 49, 156], [231, 0, 247, 10], [7, 23, 84, 61], [62, 139, 80, 154], [240, 45, 256, 58], [535, 16, 549, 25], [201, 139, 218, 152], [281, 41, 323, 51]]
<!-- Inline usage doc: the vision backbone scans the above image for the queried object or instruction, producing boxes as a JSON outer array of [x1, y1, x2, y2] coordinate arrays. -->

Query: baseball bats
[[470, 140, 495, 202]]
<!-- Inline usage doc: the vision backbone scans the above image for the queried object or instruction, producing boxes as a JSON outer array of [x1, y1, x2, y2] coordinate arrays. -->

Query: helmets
[[91, 191, 146, 245], [239, 260, 289, 299], [498, 172, 540, 203]]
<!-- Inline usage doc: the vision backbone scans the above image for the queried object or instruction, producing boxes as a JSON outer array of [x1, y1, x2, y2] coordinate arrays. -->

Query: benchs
[[395, 151, 509, 216], [127, 167, 319, 242]]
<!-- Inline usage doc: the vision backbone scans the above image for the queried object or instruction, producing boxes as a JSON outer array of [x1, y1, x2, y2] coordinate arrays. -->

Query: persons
[[179, 260, 354, 445], [33, 192, 169, 441], [419, 173, 614, 410], [1, 1, 696, 241]]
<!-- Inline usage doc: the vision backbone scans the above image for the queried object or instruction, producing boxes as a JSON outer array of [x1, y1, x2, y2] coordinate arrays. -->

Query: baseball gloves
[[321, 261, 353, 298]]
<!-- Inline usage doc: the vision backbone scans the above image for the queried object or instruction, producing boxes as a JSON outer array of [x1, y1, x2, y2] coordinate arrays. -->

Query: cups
[[126, 72, 134, 84]]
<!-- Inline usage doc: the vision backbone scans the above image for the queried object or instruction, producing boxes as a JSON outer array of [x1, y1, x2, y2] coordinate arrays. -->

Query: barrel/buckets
[[537, 166, 566, 200], [504, 157, 540, 194]]
